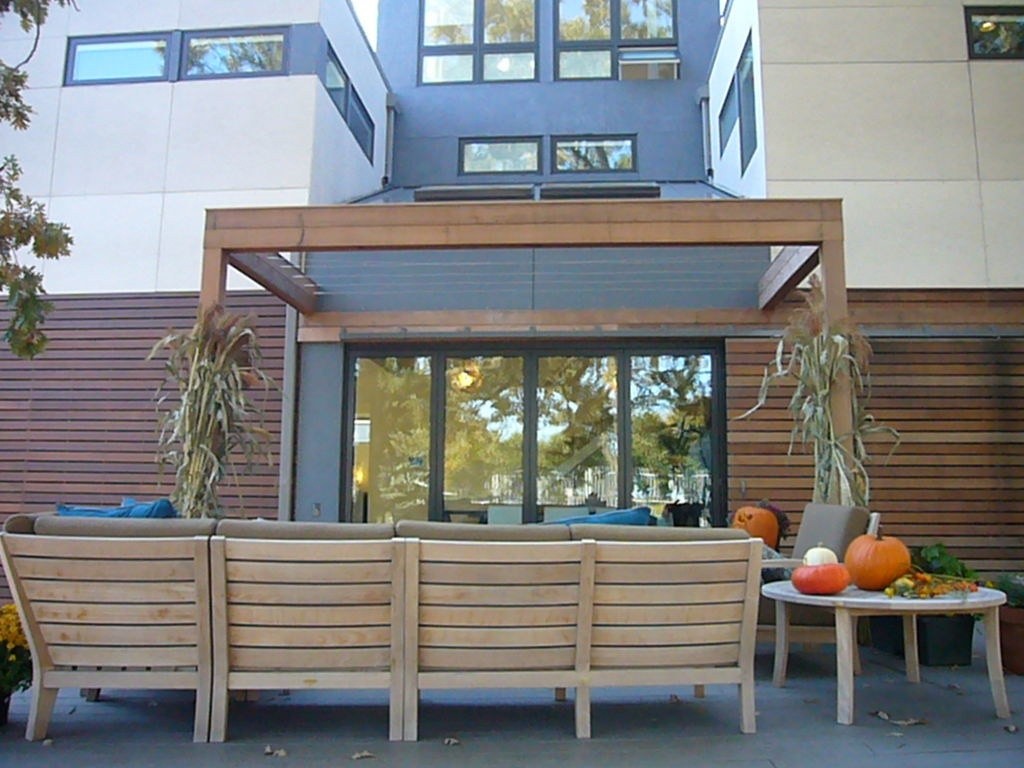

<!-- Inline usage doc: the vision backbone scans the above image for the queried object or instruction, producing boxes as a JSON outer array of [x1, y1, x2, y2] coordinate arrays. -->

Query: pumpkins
[[791, 562, 850, 595], [884, 572, 931, 597], [843, 526, 911, 590]]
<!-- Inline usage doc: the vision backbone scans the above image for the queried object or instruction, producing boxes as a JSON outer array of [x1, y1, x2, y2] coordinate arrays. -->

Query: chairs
[[1, 502, 880, 739]]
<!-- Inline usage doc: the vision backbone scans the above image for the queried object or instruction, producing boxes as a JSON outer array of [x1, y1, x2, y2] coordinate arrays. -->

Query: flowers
[[0, 603, 34, 693]]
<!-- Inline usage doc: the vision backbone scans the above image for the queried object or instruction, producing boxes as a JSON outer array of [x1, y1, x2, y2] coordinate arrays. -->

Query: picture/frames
[[965, 6, 1024, 59]]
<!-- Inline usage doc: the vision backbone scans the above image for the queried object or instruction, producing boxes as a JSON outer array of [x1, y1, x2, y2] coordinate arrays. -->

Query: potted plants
[[992, 571, 1024, 675], [873, 542, 980, 666]]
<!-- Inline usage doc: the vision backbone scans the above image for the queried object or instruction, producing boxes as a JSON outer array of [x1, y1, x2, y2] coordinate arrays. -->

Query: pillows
[[56, 498, 179, 517], [537, 505, 651, 525]]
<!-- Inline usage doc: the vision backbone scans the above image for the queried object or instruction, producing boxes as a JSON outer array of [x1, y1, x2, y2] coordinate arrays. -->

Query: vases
[[1, 688, 12, 725]]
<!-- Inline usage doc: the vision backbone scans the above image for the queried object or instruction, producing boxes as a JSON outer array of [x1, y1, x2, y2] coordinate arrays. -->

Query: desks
[[760, 577, 1010, 725]]
